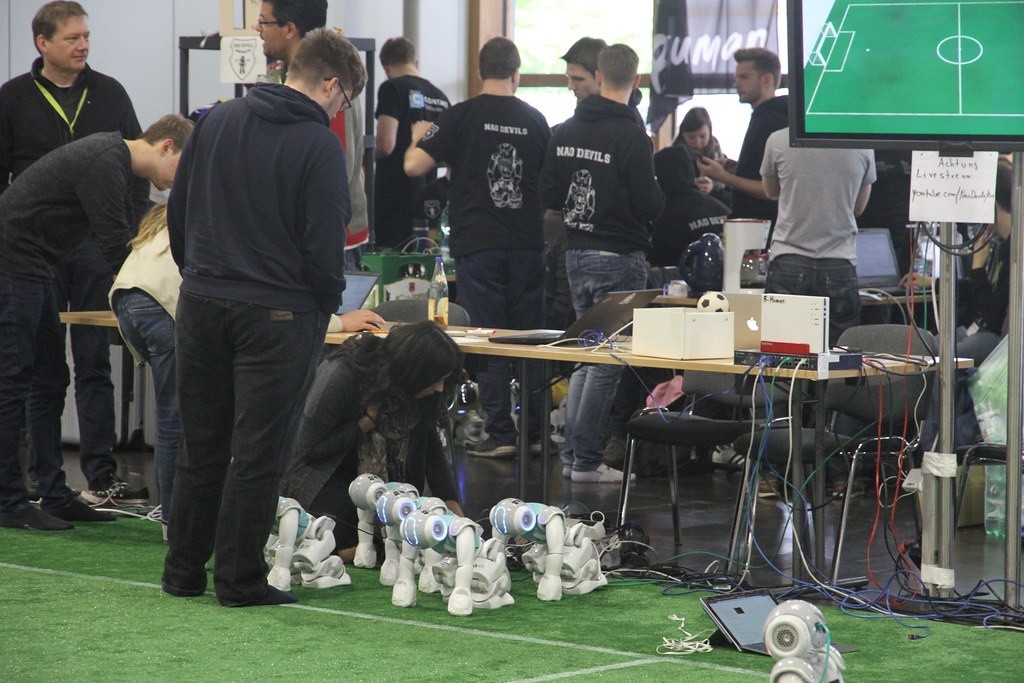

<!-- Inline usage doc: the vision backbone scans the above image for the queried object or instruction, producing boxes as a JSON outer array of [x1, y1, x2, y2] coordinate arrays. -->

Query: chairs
[[617, 367, 809, 575], [723, 324, 937, 596]]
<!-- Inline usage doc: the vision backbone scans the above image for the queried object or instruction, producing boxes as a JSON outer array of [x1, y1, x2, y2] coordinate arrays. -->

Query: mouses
[[356, 322, 381, 332]]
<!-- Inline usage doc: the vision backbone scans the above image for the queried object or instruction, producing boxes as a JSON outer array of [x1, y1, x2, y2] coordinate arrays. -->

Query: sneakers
[[81, 480, 150, 508], [466, 436, 518, 459], [530, 436, 559, 456]]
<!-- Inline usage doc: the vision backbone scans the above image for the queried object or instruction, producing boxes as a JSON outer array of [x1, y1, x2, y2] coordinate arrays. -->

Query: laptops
[[489, 288, 662, 345], [334, 270, 381, 316], [699, 588, 861, 655], [720, 292, 765, 350], [855, 228, 932, 296]]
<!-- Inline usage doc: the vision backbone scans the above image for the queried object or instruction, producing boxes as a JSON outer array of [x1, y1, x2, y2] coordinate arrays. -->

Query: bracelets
[[365, 411, 378, 426]]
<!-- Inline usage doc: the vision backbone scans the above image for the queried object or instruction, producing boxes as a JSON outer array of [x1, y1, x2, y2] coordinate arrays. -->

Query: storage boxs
[[632, 306, 735, 361]]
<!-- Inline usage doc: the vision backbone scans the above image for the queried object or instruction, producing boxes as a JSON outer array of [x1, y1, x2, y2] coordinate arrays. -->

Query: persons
[[1, 1, 1016, 608]]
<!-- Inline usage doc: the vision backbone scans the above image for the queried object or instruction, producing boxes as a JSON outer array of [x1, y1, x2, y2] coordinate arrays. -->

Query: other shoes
[[745, 469, 785, 498], [711, 442, 753, 471], [828, 475, 866, 500]]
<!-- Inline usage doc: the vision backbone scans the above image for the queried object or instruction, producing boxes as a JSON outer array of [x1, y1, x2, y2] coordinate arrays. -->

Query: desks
[[731, 290, 941, 588], [58, 311, 975, 599]]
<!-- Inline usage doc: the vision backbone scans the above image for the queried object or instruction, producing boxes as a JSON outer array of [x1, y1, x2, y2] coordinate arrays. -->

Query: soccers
[[697, 292, 729, 312]]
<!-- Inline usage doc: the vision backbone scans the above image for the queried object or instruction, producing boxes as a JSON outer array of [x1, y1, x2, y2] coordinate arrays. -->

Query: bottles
[[427, 255, 449, 330]]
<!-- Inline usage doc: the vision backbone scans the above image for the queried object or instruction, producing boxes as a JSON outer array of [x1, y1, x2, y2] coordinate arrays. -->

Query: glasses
[[322, 76, 351, 112], [254, 18, 297, 29]]
